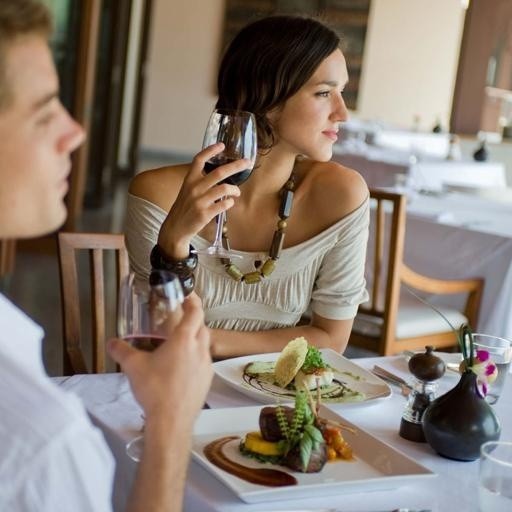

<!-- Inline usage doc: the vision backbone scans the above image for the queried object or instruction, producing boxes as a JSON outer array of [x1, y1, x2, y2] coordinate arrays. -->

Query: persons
[[121, 12, 371, 357], [0, 0, 217, 512]]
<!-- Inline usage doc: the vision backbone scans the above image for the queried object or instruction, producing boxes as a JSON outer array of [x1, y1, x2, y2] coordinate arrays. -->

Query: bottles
[[399, 345, 446, 443]]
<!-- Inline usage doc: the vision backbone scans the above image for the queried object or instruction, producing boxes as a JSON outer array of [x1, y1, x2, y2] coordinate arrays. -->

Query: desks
[[332, 111, 512, 359]]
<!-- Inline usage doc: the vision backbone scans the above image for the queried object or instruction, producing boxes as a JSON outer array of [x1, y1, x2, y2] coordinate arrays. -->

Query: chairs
[[58, 233, 148, 376]]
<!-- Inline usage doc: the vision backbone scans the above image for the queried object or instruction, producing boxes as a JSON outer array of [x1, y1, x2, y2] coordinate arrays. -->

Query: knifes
[[372, 364, 412, 394]]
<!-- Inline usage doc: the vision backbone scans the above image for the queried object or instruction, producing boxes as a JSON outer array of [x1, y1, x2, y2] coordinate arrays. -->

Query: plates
[[213, 347, 393, 409], [189, 400, 439, 505]]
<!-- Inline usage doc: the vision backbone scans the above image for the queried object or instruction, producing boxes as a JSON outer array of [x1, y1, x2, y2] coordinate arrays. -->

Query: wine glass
[[190, 107, 258, 260], [115, 268, 185, 466]]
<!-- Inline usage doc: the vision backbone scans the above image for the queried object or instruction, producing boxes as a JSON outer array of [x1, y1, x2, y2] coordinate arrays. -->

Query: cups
[[475, 441, 512, 512], [465, 332, 511, 405]]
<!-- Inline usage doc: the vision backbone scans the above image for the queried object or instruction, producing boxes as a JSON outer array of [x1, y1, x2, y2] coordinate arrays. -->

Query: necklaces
[[217, 153, 304, 285]]
